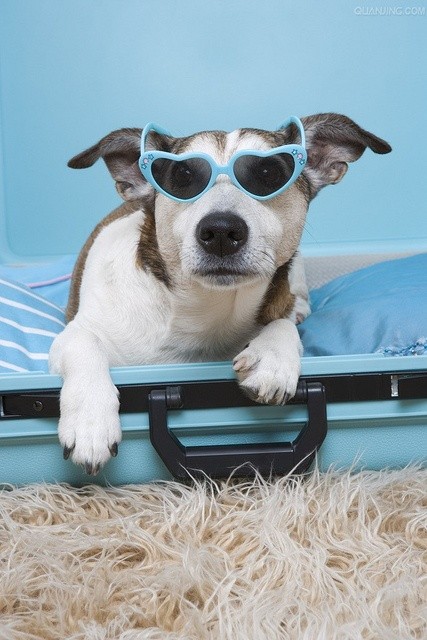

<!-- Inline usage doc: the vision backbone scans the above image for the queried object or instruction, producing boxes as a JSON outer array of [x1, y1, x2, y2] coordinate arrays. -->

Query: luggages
[[138, 115, 308, 203], [1, 249, 426, 491]]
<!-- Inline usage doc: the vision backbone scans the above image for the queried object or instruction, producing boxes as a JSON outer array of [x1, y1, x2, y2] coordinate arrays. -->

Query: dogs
[[48, 113, 393, 476]]
